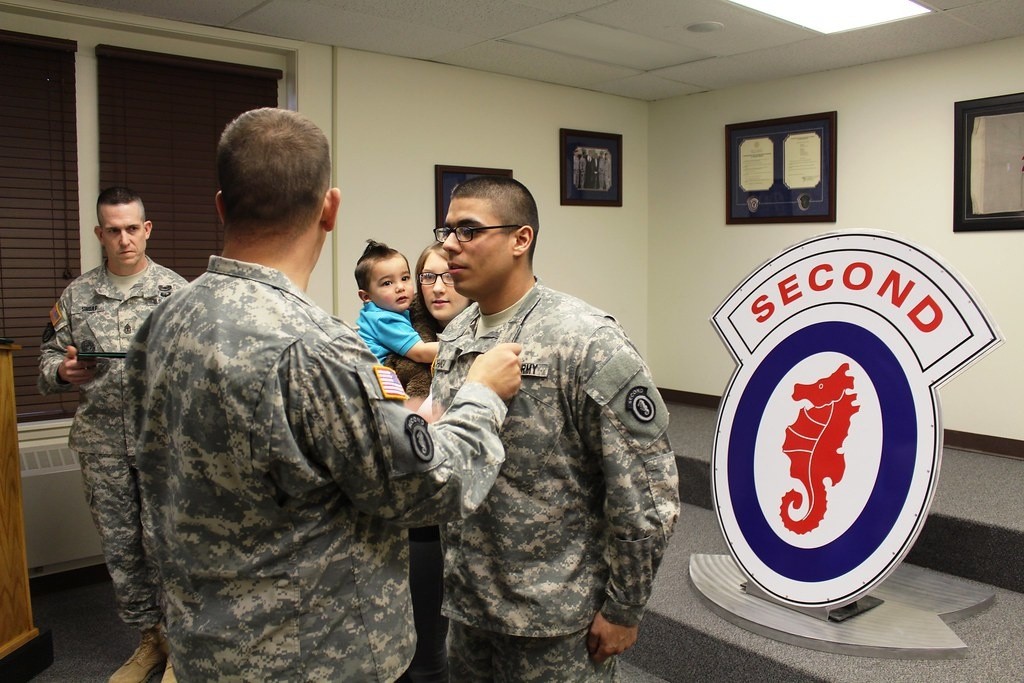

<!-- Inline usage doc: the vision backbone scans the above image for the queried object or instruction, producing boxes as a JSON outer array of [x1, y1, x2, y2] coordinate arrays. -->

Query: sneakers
[[161, 659, 177, 683], [108, 623, 170, 683]]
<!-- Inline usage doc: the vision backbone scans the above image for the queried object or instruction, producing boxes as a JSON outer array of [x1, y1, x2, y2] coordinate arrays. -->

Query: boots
[[393, 615, 449, 683]]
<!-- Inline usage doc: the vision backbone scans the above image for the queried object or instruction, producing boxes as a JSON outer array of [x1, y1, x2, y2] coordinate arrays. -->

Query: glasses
[[434, 225, 522, 242], [419, 272, 454, 284]]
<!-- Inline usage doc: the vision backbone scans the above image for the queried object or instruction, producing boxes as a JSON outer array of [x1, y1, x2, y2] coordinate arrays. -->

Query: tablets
[[78, 352, 128, 361]]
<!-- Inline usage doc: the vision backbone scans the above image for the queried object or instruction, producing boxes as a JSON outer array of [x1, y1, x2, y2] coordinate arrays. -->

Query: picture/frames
[[559, 127, 622, 206], [953, 92, 1023, 231], [435, 164, 513, 229], [726, 110, 836, 223]]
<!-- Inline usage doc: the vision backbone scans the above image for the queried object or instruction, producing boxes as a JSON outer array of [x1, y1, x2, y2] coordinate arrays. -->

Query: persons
[[573, 149, 579, 188], [354, 239, 440, 368], [594, 153, 598, 159], [431, 175, 680, 683], [599, 153, 611, 191], [33, 186, 191, 683], [125, 106, 523, 683], [598, 150, 605, 160], [577, 154, 586, 188], [584, 150, 598, 189], [384, 243, 476, 683]]
